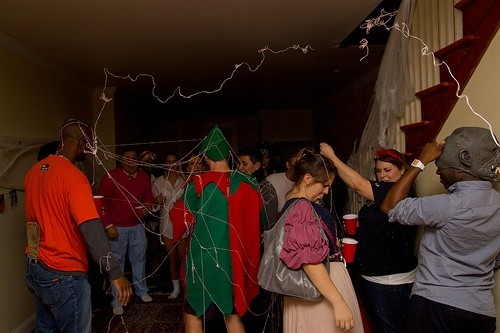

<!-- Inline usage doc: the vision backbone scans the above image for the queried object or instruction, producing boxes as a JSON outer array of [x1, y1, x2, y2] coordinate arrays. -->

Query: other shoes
[[141, 294, 152, 301], [113, 306, 124, 315]]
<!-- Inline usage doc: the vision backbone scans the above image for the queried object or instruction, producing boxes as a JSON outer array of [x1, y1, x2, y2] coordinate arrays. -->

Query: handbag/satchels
[[257, 196, 330, 302]]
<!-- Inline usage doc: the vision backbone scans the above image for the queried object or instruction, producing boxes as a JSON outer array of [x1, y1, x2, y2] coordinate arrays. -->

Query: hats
[[200, 125, 230, 162]]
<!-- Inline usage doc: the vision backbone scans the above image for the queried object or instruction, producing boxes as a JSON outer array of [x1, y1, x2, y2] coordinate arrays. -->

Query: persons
[[169, 124, 265, 333], [186, 152, 205, 177], [96, 147, 153, 316], [380, 127, 500, 333], [151, 152, 192, 300], [319, 143, 419, 333], [24, 119, 133, 333], [139, 151, 162, 289], [280, 147, 365, 333], [266, 152, 299, 213], [262, 152, 279, 178], [237, 148, 278, 333]]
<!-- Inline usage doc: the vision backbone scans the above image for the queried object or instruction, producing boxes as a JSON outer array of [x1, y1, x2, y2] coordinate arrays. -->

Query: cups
[[93, 196, 104, 211], [135, 207, 144, 219], [343, 214, 358, 235], [342, 238, 358, 263]]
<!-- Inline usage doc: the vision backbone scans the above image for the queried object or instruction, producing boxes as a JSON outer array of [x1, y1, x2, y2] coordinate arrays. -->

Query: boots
[[167, 280, 180, 299]]
